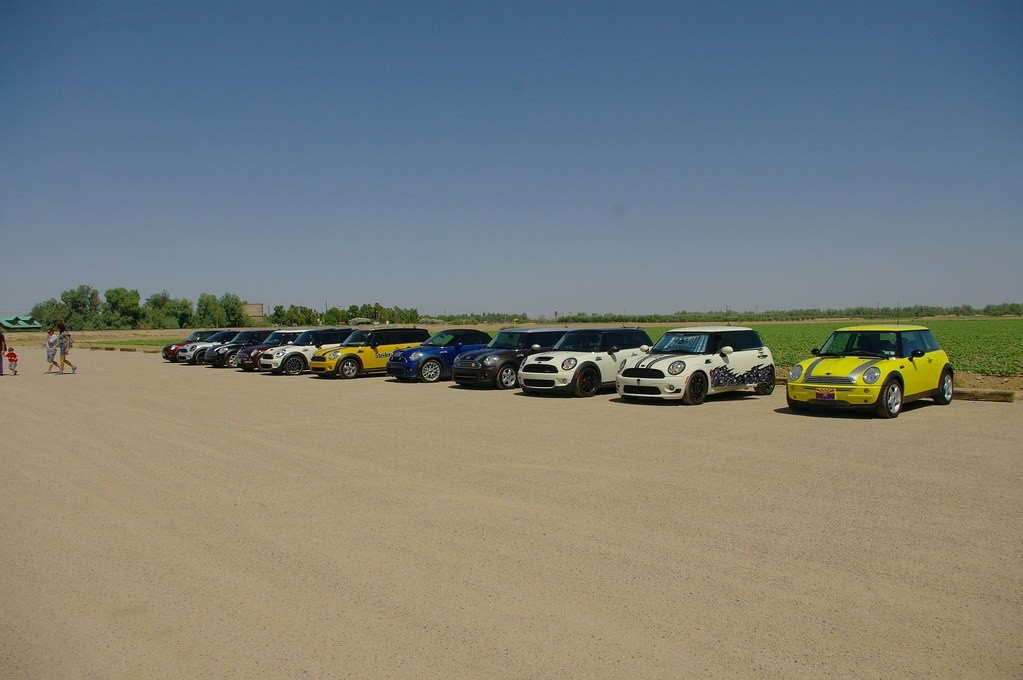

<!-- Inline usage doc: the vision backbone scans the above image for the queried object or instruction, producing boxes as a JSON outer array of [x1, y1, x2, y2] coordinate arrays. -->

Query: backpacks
[[62, 331, 74, 348]]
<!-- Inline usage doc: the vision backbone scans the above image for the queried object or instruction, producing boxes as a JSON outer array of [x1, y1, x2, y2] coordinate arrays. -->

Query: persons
[[0, 327, 18, 375], [56, 324, 78, 375], [42, 329, 65, 373]]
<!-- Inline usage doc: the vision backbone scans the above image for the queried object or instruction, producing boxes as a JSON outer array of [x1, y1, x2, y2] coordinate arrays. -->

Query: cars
[[258, 326, 369, 377], [176, 330, 241, 365], [204, 329, 299, 368], [161, 329, 223, 363], [613, 323, 778, 406], [785, 323, 955, 421], [385, 327, 513, 384], [451, 325, 603, 390], [516, 328, 655, 399], [309, 327, 434, 379], [236, 329, 312, 372]]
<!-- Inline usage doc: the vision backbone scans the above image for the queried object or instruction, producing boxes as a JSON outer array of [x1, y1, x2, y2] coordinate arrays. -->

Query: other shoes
[[72, 365, 78, 374], [55, 371, 64, 375], [43, 371, 51, 374], [11, 371, 17, 375]]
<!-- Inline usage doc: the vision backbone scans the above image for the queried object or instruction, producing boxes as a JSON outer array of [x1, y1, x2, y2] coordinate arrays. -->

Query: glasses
[[47, 331, 51, 333]]
